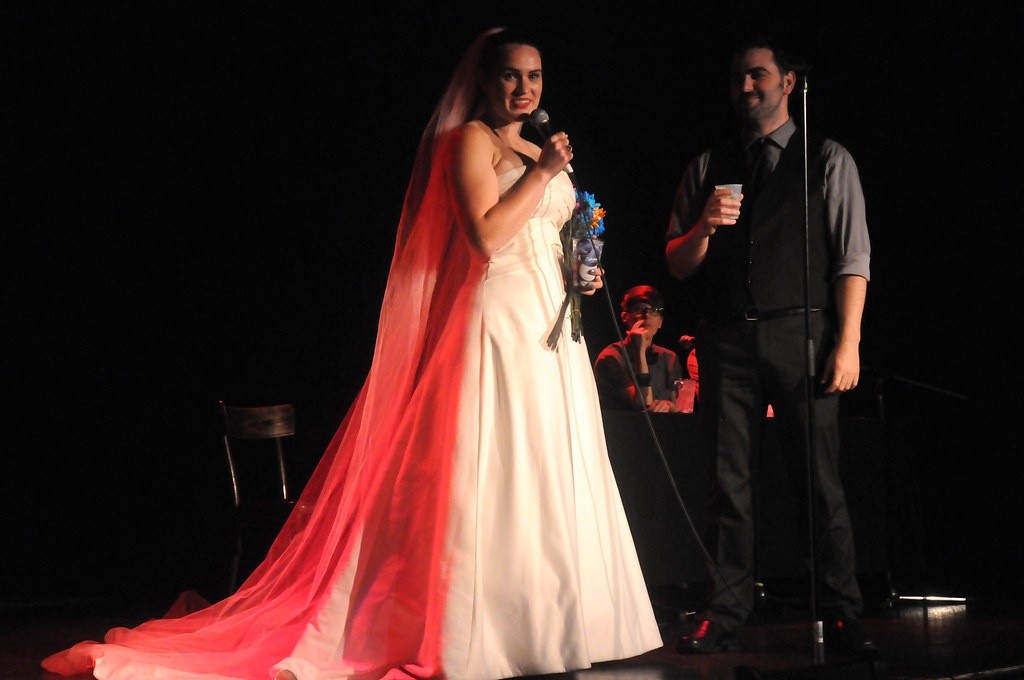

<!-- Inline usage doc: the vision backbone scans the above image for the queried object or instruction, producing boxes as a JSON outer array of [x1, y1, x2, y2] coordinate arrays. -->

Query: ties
[[747, 140, 777, 199]]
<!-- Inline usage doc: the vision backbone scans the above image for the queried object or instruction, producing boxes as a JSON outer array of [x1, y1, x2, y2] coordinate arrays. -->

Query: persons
[[595, 286, 691, 628], [274, 28, 663, 680], [666, 34, 879, 656]]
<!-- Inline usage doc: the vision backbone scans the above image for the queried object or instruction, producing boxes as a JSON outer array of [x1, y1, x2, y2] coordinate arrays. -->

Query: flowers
[[560, 184, 606, 344]]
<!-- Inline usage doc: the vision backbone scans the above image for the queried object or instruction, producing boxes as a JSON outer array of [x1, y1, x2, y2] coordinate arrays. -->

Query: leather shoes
[[824, 623, 880, 657], [678, 620, 747, 653]]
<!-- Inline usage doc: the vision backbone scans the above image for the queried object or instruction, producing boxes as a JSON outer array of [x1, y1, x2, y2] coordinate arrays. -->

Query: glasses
[[628, 302, 665, 317]]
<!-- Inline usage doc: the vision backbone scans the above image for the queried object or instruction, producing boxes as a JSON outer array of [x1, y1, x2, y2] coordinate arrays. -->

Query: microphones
[[530, 108, 578, 186]]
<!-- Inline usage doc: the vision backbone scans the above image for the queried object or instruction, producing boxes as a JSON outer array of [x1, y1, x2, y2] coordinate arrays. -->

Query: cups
[[715, 184, 743, 219], [572, 237, 604, 295], [678, 379, 695, 412]]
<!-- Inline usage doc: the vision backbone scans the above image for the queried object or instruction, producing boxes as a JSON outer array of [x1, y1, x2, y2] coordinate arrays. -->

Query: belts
[[744, 308, 822, 321]]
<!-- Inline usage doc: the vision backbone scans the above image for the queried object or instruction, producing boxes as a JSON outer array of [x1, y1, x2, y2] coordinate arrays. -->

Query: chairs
[[217, 398, 302, 601]]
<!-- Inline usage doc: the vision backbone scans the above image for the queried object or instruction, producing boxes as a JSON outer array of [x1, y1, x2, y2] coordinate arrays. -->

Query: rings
[[566, 145, 572, 152]]
